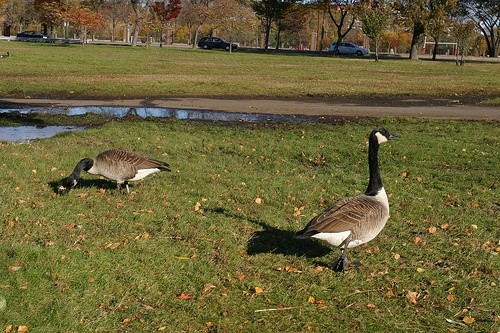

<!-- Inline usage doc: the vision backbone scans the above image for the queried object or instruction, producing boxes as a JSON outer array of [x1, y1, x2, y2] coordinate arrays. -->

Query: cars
[[328, 42, 369, 56], [17, 30, 48, 42], [198, 37, 237, 50]]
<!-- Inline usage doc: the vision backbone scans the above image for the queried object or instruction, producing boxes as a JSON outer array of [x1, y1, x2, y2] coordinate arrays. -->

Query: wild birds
[[58, 149, 171, 196], [297, 127, 401, 271], [1, 52, 9, 59]]
